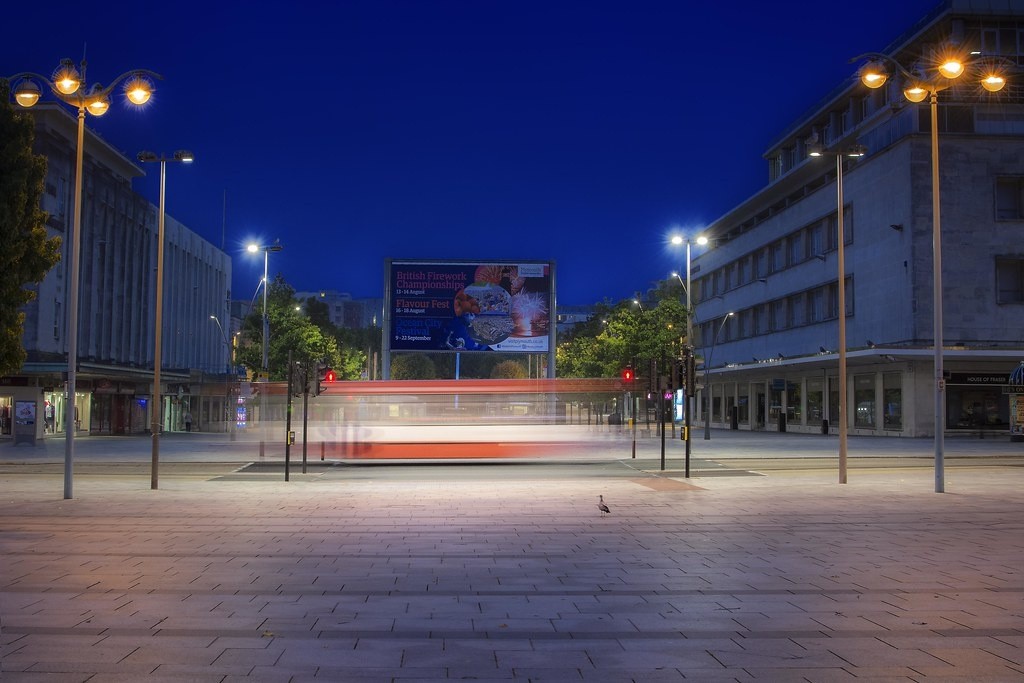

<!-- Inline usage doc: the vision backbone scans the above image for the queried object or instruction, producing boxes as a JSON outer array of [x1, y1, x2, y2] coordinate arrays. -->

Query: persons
[[979, 404, 987, 439], [46, 402, 55, 432], [185, 412, 192, 432]]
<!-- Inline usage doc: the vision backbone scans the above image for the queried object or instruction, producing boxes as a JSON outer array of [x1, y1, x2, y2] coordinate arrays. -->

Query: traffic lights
[[620, 346, 634, 382], [321, 364, 336, 382]]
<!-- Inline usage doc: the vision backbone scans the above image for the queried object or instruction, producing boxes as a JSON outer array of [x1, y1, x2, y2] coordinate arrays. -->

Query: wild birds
[[596, 494, 612, 519]]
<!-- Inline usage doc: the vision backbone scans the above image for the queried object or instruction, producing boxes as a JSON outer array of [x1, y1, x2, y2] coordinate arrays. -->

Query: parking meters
[[628, 418, 633, 430], [288, 430, 296, 446], [680, 426, 688, 441]]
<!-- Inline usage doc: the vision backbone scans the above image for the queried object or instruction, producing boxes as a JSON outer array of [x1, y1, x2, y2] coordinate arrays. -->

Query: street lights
[[670, 234, 710, 477], [246, 243, 285, 460], [138, 150, 196, 492], [849, 43, 1013, 491], [210, 277, 267, 440], [672, 273, 735, 441], [807, 146, 865, 485], [1, 58, 167, 500]]
